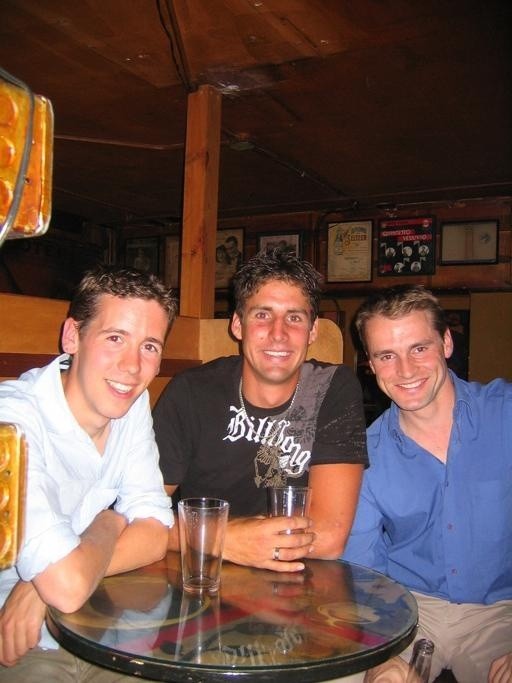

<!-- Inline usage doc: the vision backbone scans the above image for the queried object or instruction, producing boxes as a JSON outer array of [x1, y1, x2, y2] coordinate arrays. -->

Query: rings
[[273, 584, 280, 593], [274, 548, 280, 561]]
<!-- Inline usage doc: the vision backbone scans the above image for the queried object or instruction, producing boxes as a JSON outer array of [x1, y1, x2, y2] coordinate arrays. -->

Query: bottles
[[405, 638, 433, 683]]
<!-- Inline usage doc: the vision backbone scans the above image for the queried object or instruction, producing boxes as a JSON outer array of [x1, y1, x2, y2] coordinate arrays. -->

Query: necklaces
[[237, 374, 302, 440]]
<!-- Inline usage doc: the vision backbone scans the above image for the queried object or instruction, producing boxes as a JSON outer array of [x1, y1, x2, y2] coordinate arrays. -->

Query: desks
[[44, 555, 424, 683]]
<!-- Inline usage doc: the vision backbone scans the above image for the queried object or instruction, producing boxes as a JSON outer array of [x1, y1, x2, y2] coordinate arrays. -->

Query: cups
[[267, 484, 309, 566], [176, 498, 228, 594]]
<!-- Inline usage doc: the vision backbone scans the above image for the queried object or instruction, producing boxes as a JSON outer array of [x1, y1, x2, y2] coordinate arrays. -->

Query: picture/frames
[[158, 233, 181, 294], [213, 226, 246, 294], [256, 230, 302, 262], [377, 217, 436, 277], [438, 217, 498, 266], [324, 220, 374, 283], [124, 237, 164, 280]]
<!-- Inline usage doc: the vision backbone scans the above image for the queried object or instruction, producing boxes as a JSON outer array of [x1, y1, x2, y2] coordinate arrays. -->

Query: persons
[[1, 266, 179, 682], [331, 284, 510, 683], [212, 245, 226, 265], [224, 235, 242, 261], [154, 550, 364, 659], [140, 245, 372, 573]]
